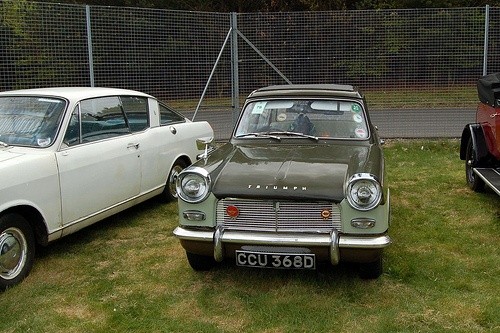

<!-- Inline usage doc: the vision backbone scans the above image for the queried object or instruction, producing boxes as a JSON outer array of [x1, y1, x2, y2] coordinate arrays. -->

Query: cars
[[459, 72, 500, 201], [0, 86, 215, 290], [172, 84, 391, 278]]
